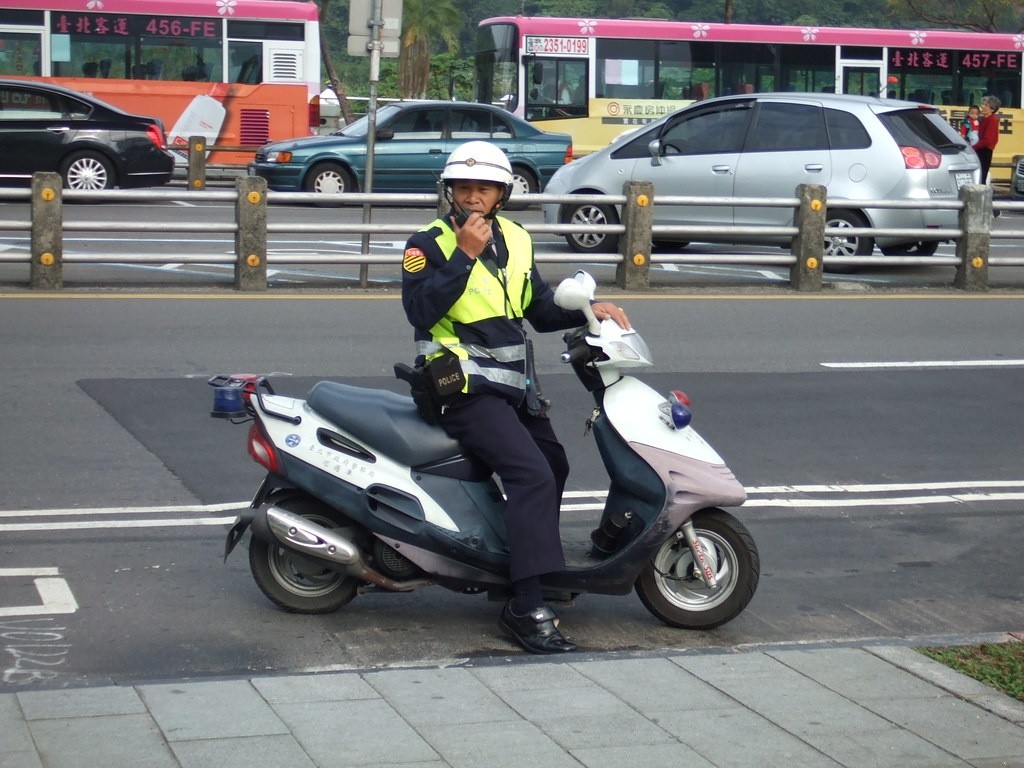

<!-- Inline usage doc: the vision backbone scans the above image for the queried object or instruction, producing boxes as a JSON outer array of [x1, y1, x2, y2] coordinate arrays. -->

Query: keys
[[582, 408, 600, 437]]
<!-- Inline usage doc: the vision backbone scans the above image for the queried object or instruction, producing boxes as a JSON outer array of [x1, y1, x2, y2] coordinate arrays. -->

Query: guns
[[392, 362, 439, 426]]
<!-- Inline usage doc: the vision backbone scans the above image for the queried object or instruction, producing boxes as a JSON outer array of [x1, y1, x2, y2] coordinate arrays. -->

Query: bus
[[0, 0, 320, 193], [472, 14, 1024, 188]]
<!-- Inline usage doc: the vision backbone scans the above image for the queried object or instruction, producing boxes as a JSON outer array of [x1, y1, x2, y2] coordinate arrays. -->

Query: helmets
[[440, 140, 513, 217]]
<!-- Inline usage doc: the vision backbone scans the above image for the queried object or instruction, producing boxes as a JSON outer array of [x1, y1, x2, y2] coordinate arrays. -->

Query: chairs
[[646, 82, 1015, 102], [33, 58, 264, 85]]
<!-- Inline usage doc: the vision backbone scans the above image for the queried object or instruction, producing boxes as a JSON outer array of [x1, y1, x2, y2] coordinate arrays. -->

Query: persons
[[959, 95, 1002, 219], [399, 139, 636, 655]]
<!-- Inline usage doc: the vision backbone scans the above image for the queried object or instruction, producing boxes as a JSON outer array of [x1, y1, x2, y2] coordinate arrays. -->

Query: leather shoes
[[497, 598, 577, 654]]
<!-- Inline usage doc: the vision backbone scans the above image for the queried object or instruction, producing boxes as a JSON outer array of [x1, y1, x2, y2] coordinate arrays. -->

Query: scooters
[[208, 267, 762, 630]]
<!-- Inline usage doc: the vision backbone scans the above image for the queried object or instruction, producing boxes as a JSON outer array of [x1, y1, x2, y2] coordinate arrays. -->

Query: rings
[[618, 307, 622, 313]]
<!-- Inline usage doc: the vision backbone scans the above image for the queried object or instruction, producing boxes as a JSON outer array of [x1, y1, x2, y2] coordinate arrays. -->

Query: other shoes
[[993, 209, 1002, 219]]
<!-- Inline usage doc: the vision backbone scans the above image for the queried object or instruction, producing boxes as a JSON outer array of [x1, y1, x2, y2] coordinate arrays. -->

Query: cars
[[0, 78, 176, 205], [1009, 154, 1024, 197], [252, 100, 573, 208], [542, 91, 982, 274]]
